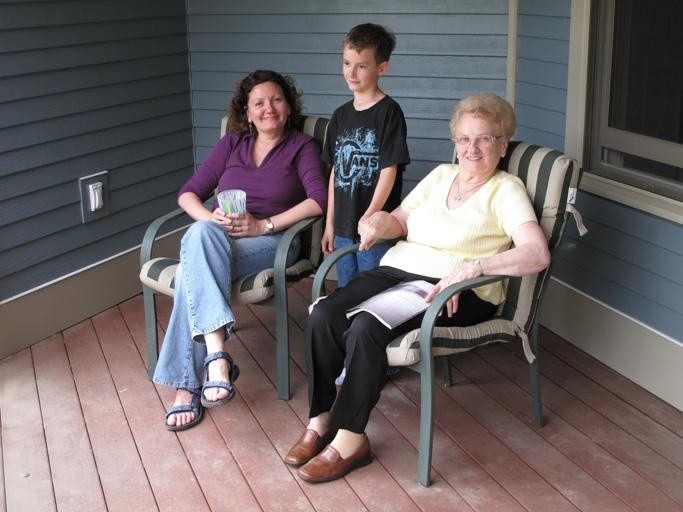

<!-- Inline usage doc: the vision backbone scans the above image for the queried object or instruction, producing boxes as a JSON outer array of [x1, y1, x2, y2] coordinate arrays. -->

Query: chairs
[[312, 141, 587, 489], [140, 112, 331, 400]]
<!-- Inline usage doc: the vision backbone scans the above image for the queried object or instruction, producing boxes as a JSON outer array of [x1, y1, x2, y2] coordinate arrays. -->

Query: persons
[[151, 67, 327, 431], [285, 91, 553, 484], [318, 22, 414, 288]]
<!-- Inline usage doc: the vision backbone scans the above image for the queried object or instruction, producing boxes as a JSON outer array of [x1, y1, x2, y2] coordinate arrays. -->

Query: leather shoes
[[296, 430, 373, 483], [284, 412, 339, 465]]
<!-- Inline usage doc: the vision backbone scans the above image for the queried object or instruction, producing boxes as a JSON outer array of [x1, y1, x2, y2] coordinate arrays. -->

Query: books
[[346, 280, 436, 331]]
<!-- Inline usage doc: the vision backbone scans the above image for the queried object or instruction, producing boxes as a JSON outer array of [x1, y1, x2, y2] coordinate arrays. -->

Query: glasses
[[454, 133, 505, 146]]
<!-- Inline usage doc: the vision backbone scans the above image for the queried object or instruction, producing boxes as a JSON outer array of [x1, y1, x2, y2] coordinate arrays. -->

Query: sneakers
[[335, 358, 403, 386]]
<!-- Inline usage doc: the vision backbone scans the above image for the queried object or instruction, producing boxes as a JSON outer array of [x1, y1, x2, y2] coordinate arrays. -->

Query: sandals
[[164, 385, 204, 431], [200, 350, 240, 408]]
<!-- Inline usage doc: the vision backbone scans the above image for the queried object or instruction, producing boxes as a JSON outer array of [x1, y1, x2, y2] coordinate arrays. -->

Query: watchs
[[473, 257, 486, 277], [265, 217, 278, 235]]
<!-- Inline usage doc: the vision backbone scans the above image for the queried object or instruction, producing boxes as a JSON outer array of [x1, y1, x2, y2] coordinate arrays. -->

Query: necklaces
[[453, 175, 481, 203]]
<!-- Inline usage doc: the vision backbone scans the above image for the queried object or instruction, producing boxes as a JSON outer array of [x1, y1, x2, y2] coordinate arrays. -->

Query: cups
[[217, 190, 247, 217]]
